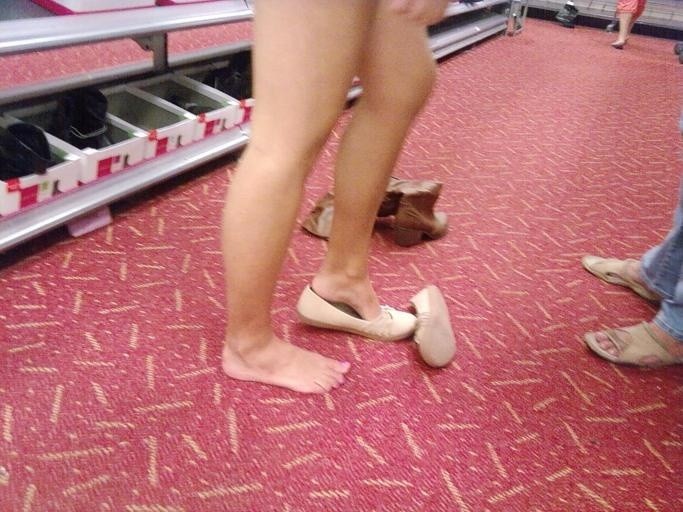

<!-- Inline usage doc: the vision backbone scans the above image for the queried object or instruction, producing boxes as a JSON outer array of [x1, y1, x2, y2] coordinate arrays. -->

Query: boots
[[51, 88, 111, 149], [2, 121, 52, 182], [376, 176, 448, 246], [301, 188, 335, 240]]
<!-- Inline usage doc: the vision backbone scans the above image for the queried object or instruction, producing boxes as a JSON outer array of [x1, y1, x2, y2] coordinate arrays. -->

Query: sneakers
[[408, 285, 456, 367]]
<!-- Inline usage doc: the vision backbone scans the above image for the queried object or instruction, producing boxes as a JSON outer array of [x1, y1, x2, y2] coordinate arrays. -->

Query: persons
[[580, 178, 682, 369], [610, 0, 648, 49], [220, 1, 453, 395]]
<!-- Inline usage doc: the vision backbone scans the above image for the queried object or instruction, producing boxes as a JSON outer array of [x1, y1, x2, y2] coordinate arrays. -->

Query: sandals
[[585, 321, 683, 366], [580, 253, 663, 301]]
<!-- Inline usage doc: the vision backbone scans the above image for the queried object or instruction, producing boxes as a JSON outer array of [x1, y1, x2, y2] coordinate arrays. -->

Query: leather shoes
[[295, 284, 418, 340]]
